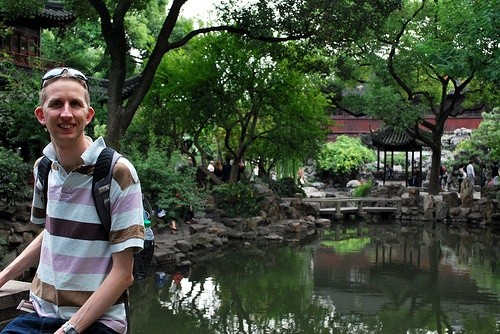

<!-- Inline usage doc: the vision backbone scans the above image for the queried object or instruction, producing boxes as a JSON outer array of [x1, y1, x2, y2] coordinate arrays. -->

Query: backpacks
[[37, 147, 154, 280]]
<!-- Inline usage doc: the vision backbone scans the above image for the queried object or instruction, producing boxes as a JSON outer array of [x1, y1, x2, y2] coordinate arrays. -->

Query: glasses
[[41, 68, 90, 92]]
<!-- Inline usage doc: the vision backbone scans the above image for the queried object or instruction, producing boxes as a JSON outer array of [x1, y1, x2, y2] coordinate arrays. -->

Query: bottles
[[143, 220, 154, 240]]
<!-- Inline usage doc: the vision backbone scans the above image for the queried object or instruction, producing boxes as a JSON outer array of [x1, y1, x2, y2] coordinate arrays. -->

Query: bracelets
[[59, 318, 80, 334]]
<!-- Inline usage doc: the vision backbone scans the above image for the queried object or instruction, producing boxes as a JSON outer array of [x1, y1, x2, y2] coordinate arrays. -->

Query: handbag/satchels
[[457, 170, 463, 177]]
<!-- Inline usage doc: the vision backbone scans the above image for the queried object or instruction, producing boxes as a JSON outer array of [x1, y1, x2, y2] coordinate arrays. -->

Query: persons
[[1, 67, 145, 334], [187, 154, 500, 195]]
